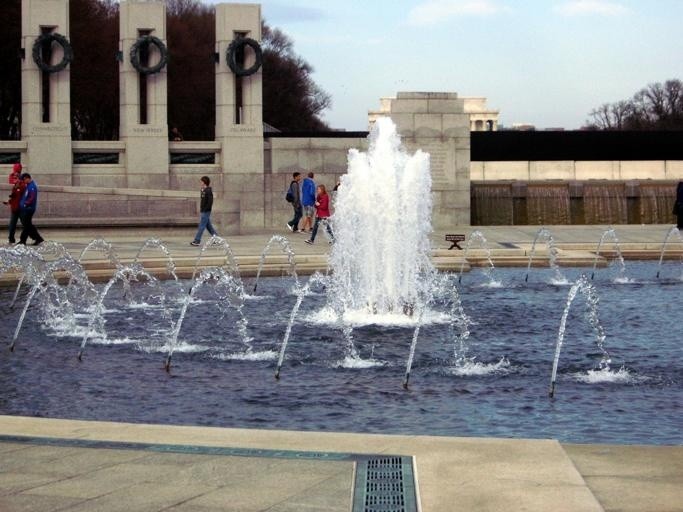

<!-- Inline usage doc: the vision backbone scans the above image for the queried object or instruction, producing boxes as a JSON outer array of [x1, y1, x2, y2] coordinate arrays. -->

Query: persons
[[305, 185, 335, 245], [190, 176, 219, 246], [17, 173, 44, 245], [168, 128, 183, 141], [3, 173, 26, 242], [300, 172, 316, 232], [287, 172, 303, 232]]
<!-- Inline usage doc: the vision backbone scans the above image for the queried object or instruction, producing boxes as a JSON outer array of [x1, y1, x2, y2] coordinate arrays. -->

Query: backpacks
[[285, 181, 298, 202]]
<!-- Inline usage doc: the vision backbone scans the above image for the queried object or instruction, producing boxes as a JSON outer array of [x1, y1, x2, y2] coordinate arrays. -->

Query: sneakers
[[292, 228, 301, 233], [190, 240, 200, 246], [286, 221, 292, 231], [305, 238, 314, 244], [14, 241, 26, 247], [329, 239, 334, 245], [31, 237, 45, 246]]
